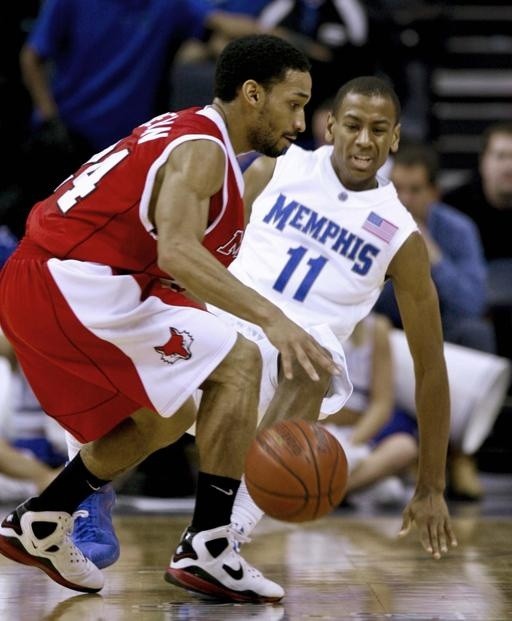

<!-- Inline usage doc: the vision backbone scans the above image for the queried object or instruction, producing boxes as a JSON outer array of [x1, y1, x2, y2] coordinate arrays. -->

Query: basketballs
[[246, 419, 349, 524]]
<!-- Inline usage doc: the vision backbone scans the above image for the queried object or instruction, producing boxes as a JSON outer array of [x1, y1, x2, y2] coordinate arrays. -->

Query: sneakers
[[164, 526, 285, 605], [0, 497, 105, 593], [72, 485, 120, 569]]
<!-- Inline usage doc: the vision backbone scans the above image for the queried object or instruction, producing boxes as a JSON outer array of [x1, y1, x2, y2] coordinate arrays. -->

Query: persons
[[67, 74, 457, 571], [1, 34, 339, 607], [1, 0, 512, 514]]
